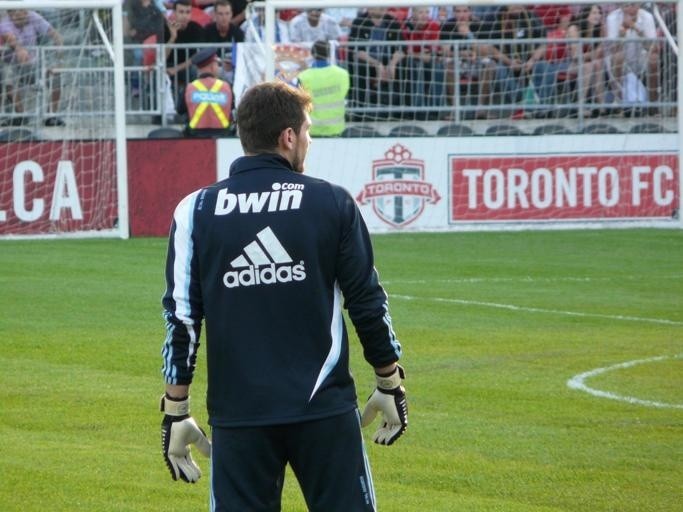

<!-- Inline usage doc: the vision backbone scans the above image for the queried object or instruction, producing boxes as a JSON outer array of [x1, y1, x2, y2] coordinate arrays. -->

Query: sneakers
[[45, 117, 66, 125], [1, 117, 30, 126]]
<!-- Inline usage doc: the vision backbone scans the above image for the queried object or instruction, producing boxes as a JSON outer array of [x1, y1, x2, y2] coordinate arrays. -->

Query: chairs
[[339, 123, 675, 136], [147, 127, 184, 138], [0, 128, 38, 141]]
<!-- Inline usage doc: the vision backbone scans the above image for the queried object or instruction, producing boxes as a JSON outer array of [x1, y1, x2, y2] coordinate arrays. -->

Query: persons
[[176, 46, 237, 136], [0, 9, 67, 126], [291, 40, 350, 137], [159, 81, 408, 512], [85, 0, 680, 124]]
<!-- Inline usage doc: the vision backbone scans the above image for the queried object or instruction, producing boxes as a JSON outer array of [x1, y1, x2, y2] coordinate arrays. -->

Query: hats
[[191, 46, 217, 68]]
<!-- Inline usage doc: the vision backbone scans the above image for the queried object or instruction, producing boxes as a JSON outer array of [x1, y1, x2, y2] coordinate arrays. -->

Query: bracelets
[[159, 395, 190, 418], [374, 365, 406, 393]]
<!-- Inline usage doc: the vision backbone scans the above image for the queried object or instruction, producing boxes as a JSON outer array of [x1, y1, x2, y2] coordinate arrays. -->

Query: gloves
[[159, 394, 211, 484], [360, 363, 408, 446]]
